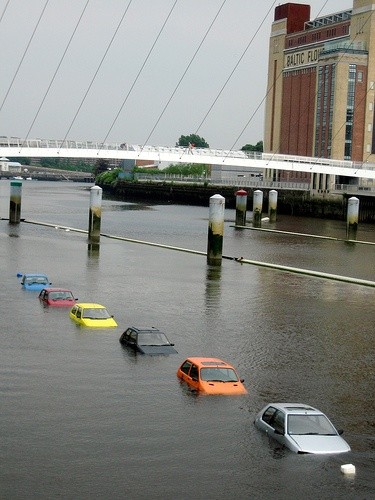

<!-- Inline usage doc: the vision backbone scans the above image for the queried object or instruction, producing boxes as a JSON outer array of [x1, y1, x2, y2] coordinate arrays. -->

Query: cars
[[17, 272, 52, 292], [38, 288, 78, 309], [176, 357, 249, 396], [69, 303, 118, 330], [119, 326, 179, 357], [254, 403, 351, 455]]
